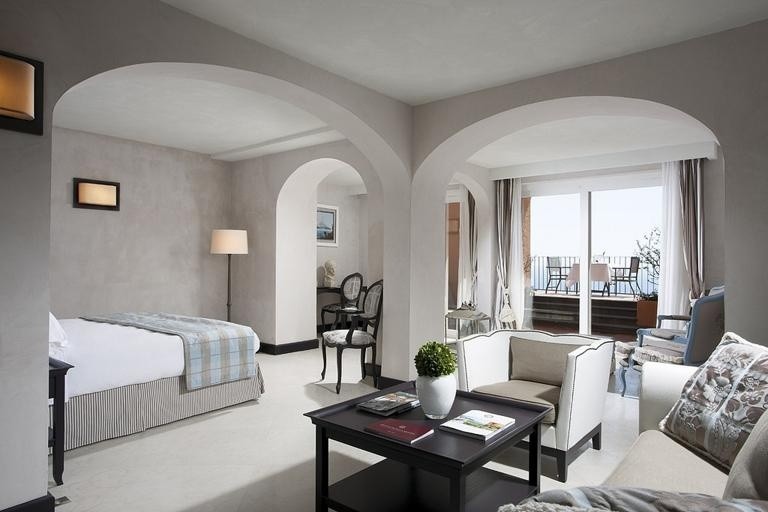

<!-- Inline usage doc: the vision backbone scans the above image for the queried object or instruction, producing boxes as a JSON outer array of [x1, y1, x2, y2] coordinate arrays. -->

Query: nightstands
[[47, 356, 74, 487]]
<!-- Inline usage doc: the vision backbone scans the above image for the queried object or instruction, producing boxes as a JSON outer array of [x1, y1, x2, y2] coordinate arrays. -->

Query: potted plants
[[413, 341, 458, 421]]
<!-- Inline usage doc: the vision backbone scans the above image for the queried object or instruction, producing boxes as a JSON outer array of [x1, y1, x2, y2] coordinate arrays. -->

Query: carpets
[[607, 362, 640, 398]]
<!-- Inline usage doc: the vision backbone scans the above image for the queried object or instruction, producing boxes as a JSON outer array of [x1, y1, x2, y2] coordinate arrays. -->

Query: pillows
[[507, 335, 589, 388], [659, 332, 767, 475], [47, 313, 69, 350], [717, 402, 767, 502]]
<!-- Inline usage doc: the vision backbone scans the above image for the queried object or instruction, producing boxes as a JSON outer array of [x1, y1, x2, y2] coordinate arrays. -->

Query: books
[[355, 390, 421, 417], [366, 418, 436, 445], [436, 410, 517, 441]]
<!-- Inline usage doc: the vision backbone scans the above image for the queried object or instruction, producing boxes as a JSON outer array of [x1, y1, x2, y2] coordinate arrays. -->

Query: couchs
[[497, 357, 767, 512]]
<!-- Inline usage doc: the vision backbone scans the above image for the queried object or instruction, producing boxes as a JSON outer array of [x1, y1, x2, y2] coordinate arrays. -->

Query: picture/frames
[[315, 203, 339, 248]]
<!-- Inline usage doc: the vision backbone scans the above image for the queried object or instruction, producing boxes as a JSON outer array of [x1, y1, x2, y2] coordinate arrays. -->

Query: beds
[[44, 309, 265, 459]]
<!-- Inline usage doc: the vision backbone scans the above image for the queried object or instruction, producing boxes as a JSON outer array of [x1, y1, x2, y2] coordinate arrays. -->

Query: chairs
[[320, 278, 382, 395], [453, 328, 615, 486], [614, 293, 726, 399], [606, 256, 643, 298], [542, 254, 578, 296], [320, 272, 364, 331]]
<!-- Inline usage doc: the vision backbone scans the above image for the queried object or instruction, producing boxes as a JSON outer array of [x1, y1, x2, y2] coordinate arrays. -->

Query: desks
[[564, 262, 611, 298], [316, 286, 367, 323]]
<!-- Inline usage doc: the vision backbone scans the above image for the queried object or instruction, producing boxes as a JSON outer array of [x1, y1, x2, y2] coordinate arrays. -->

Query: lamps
[[71, 175, 121, 212], [1, 49, 45, 138], [442, 301, 491, 352], [209, 228, 250, 322]]
